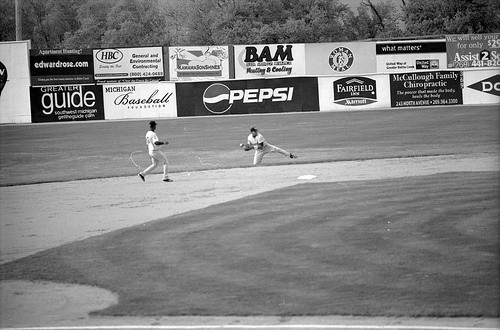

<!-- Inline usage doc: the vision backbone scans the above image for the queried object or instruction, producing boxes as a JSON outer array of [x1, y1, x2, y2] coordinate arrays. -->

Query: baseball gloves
[[243, 144, 254, 151]]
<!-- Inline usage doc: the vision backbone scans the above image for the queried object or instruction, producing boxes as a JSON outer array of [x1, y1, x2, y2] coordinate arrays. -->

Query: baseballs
[[240, 144, 243, 147]]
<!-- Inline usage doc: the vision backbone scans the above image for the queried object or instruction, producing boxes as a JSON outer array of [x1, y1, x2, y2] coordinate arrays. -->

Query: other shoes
[[138, 173, 145, 181], [291, 155, 297, 158], [164, 179, 173, 182]]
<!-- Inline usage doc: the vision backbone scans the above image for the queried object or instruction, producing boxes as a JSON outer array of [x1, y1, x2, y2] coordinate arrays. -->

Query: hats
[[149, 121, 157, 125], [250, 127, 257, 131]]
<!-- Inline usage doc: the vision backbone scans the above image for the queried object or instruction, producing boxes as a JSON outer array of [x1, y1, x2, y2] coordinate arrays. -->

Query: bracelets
[[254, 145, 258, 149]]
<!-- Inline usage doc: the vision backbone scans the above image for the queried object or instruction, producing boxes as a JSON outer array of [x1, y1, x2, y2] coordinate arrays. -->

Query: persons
[[240, 127, 297, 165], [138, 121, 173, 182]]
